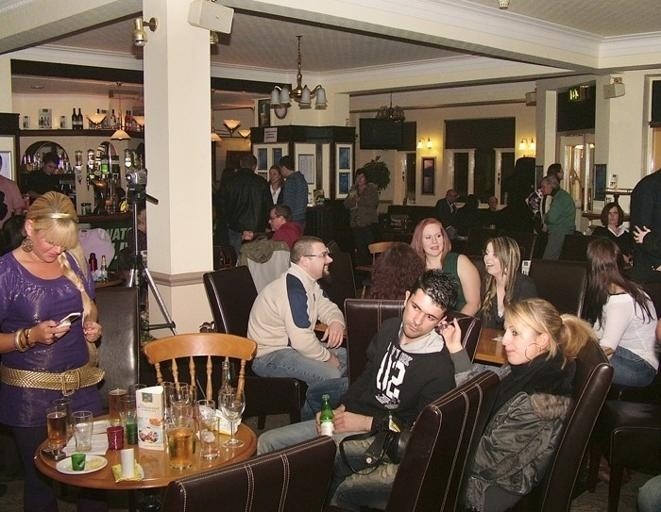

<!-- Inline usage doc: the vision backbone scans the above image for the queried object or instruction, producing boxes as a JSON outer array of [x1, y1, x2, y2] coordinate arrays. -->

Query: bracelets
[[14, 328, 35, 353]]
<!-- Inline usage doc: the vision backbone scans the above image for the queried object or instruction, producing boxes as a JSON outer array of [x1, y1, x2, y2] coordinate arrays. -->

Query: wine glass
[[217, 388, 245, 449], [162, 381, 205, 445]]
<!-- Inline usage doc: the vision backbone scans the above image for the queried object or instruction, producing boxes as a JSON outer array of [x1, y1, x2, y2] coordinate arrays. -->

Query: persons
[[637, 475, 660, 511], [247, 237, 348, 423], [213, 154, 576, 331], [130, 198, 148, 269], [0, 156, 28, 230], [22, 152, 62, 206], [0, 216, 27, 257], [580, 170, 660, 388], [1, 192, 107, 512], [329, 297, 593, 511], [257, 269, 460, 511]]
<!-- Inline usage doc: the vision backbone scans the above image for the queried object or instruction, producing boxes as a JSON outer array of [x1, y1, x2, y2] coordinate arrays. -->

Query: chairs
[[511, 333, 616, 512], [161, 434, 338, 511], [144, 332, 259, 419], [382, 368, 502, 511], [204, 264, 309, 428], [343, 297, 407, 391], [444, 311, 484, 367], [359, 233, 661, 327], [583, 394, 660, 511]]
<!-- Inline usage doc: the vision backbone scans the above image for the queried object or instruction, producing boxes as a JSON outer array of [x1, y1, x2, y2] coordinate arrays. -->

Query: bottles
[[123, 149, 140, 170], [100, 255, 107, 282], [100, 151, 120, 175], [19, 150, 94, 174], [87, 253, 97, 273], [24, 116, 28, 128], [69, 106, 134, 131], [40, 109, 51, 128], [609, 174, 617, 190], [318, 393, 336, 439], [81, 202, 92, 217], [60, 116, 66, 128]]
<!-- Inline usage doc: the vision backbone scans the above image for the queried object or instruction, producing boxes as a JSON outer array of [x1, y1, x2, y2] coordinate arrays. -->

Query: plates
[[55, 455, 107, 476]]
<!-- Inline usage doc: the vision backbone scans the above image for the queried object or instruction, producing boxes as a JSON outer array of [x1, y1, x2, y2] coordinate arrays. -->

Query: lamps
[[86, 87, 145, 140], [518, 138, 537, 151], [377, 92, 406, 128], [211, 120, 251, 142], [133, 17, 158, 47], [270, 36, 327, 106], [416, 138, 432, 149]]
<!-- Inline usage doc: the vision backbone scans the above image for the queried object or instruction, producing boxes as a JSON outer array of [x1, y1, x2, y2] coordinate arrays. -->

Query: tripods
[[122, 263, 206, 399]]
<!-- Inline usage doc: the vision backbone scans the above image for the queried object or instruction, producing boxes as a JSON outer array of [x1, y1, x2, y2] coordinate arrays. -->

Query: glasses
[[303, 247, 330, 258]]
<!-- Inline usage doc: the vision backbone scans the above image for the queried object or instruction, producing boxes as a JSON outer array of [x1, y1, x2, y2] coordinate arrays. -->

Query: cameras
[[125, 169, 147, 185]]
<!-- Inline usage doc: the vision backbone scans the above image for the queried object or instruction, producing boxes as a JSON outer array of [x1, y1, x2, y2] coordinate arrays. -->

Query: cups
[[126, 423, 138, 445], [164, 426, 195, 471], [107, 425, 125, 451], [120, 447, 135, 478], [106, 385, 137, 425], [200, 399, 217, 417], [46, 404, 67, 457], [71, 410, 94, 452], [201, 416, 222, 458], [70, 453, 86, 470]]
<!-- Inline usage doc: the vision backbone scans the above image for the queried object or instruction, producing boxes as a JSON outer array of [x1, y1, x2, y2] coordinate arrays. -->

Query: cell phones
[[54, 312, 81, 327]]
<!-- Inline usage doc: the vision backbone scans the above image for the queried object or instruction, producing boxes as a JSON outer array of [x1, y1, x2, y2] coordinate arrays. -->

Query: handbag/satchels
[[338, 409, 412, 476]]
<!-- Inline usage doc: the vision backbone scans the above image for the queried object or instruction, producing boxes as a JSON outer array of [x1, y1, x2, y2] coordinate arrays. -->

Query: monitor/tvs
[[359, 118, 403, 149]]
